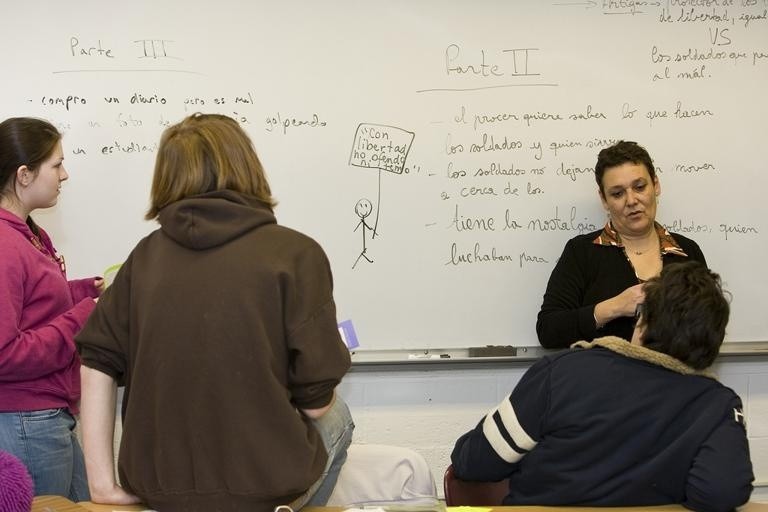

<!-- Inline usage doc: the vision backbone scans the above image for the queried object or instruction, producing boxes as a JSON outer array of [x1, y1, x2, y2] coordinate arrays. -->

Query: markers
[[409, 353, 451, 359]]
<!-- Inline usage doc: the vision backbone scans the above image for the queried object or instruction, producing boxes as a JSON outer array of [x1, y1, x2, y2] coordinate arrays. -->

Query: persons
[[0, 116, 110, 500], [534, 137, 706, 352], [74, 114, 356, 510], [447, 260, 755, 510]]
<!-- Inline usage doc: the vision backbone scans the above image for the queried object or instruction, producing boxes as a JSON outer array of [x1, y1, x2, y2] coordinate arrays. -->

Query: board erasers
[[468, 345, 518, 358]]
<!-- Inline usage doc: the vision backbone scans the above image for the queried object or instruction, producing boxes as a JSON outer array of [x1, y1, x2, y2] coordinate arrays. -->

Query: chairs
[[442, 464, 510, 507]]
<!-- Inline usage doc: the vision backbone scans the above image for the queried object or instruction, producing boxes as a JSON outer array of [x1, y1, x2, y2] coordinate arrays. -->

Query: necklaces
[[623, 244, 658, 255]]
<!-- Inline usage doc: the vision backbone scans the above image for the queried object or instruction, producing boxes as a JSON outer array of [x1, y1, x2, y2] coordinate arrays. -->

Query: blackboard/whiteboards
[[0, 0, 768, 366]]
